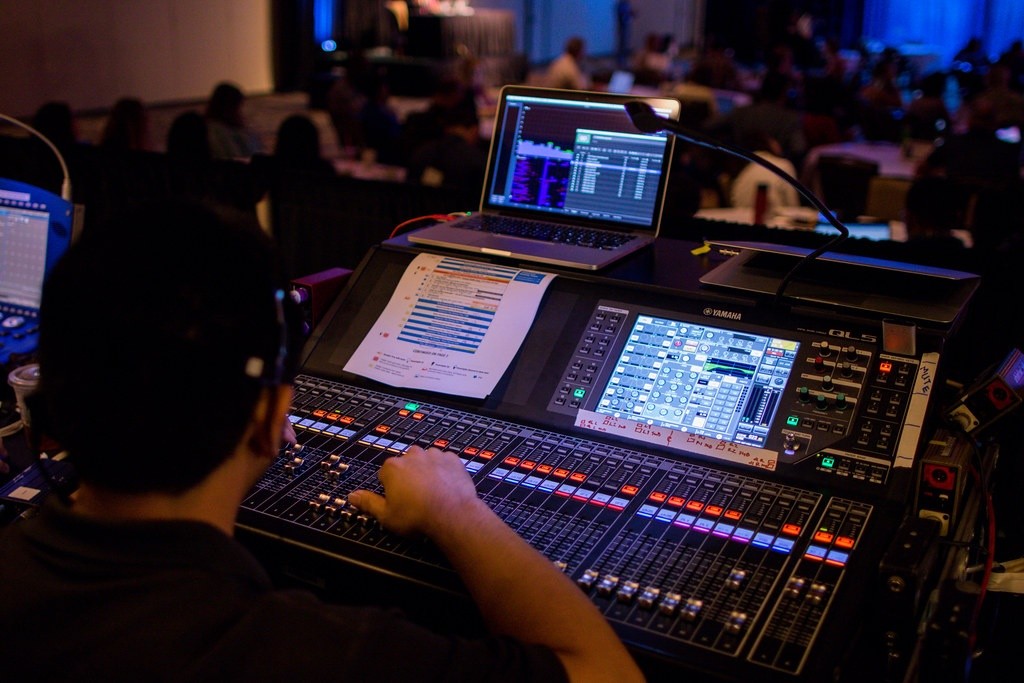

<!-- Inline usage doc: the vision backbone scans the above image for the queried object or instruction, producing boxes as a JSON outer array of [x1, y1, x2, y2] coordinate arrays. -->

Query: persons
[[0, 203, 645, 683], [10, 13, 1024, 330]]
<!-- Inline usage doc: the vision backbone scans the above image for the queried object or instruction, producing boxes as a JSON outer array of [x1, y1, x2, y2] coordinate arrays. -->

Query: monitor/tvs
[[0, 205, 50, 311], [595, 312, 802, 448]]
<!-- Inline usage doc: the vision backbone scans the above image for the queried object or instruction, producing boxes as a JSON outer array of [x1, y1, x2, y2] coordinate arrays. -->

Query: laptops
[[409, 85, 682, 270]]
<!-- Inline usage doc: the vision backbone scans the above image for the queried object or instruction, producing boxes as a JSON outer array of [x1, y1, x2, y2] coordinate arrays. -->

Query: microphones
[[624, 97, 849, 326]]
